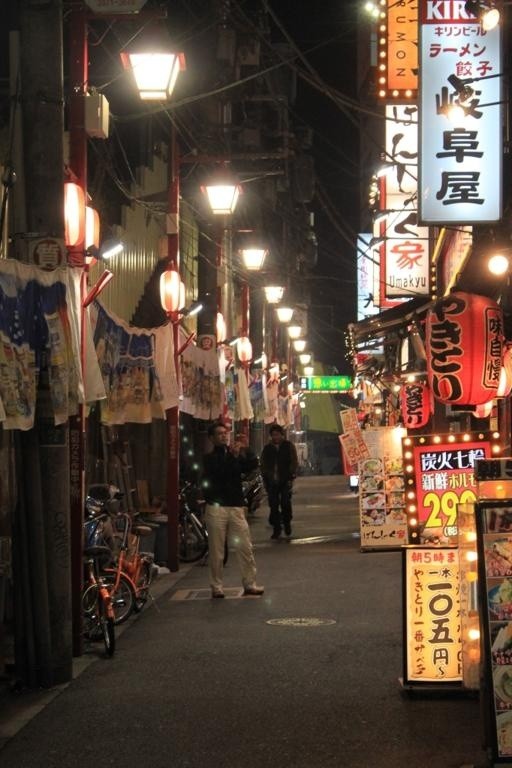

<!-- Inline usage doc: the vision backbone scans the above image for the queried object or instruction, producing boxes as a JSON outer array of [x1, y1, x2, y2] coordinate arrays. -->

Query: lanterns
[[425, 291, 504, 413], [401, 381, 431, 428]]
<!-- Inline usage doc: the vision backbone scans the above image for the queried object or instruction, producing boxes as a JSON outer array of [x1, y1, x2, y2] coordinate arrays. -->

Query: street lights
[[58, 0, 188, 571], [234, 232, 316, 457], [154, 98, 242, 569]]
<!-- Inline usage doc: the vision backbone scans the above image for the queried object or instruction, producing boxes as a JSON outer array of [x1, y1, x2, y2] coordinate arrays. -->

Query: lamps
[[158, 263, 186, 323], [216, 313, 307, 411], [61, 176, 103, 268], [116, 16, 190, 102], [262, 280, 313, 378], [236, 234, 272, 274], [200, 161, 243, 215]]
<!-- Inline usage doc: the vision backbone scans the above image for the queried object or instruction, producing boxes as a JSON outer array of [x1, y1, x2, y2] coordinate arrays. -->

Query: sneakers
[[212, 587, 224, 597], [245, 586, 264, 593]]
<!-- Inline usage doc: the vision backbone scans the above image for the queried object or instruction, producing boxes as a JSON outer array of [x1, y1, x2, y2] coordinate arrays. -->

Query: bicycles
[[76, 467, 265, 658], [298, 450, 322, 475]]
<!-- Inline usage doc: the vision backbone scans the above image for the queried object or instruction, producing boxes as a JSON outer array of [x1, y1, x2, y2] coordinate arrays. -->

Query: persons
[[261, 425, 298, 538], [202, 423, 265, 598]]
